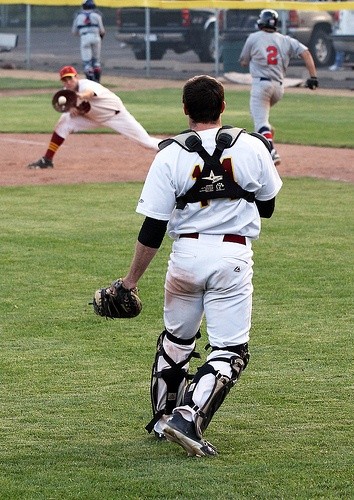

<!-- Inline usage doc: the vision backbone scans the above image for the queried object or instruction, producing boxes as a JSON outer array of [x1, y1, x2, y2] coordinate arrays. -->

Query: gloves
[[307, 76, 320, 90]]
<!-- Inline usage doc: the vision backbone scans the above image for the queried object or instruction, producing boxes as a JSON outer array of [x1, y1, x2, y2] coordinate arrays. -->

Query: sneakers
[[161, 413, 217, 458]]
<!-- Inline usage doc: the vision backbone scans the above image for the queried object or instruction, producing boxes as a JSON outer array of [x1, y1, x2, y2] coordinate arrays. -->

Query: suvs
[[114, 6, 219, 64], [207, 0, 337, 68]]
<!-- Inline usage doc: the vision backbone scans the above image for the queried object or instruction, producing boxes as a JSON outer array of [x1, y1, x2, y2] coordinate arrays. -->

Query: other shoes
[[28, 157, 54, 169], [85, 69, 100, 83], [272, 150, 281, 165]]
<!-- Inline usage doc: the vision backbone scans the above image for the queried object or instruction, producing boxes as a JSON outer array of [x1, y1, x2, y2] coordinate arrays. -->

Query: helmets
[[256, 10, 279, 29]]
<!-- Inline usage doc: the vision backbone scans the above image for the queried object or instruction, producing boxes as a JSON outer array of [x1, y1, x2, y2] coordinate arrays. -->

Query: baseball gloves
[[50, 88, 78, 113], [93, 279, 143, 318]]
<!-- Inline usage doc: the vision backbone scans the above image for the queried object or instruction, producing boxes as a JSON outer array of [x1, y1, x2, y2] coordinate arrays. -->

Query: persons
[[27, 65, 164, 170], [329, 10, 345, 72], [72, 0, 105, 84], [239, 10, 318, 167], [89, 75, 282, 458]]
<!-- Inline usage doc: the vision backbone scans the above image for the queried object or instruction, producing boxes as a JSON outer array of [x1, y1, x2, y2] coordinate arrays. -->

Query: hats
[[60, 65, 77, 79], [83, 0, 96, 9]]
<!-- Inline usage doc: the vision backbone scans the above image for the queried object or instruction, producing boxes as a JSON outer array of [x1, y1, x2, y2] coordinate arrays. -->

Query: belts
[[178, 232, 247, 246], [260, 77, 283, 85]]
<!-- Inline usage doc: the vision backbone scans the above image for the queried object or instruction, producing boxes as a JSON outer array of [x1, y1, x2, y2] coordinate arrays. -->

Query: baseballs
[[59, 96, 66, 104]]
